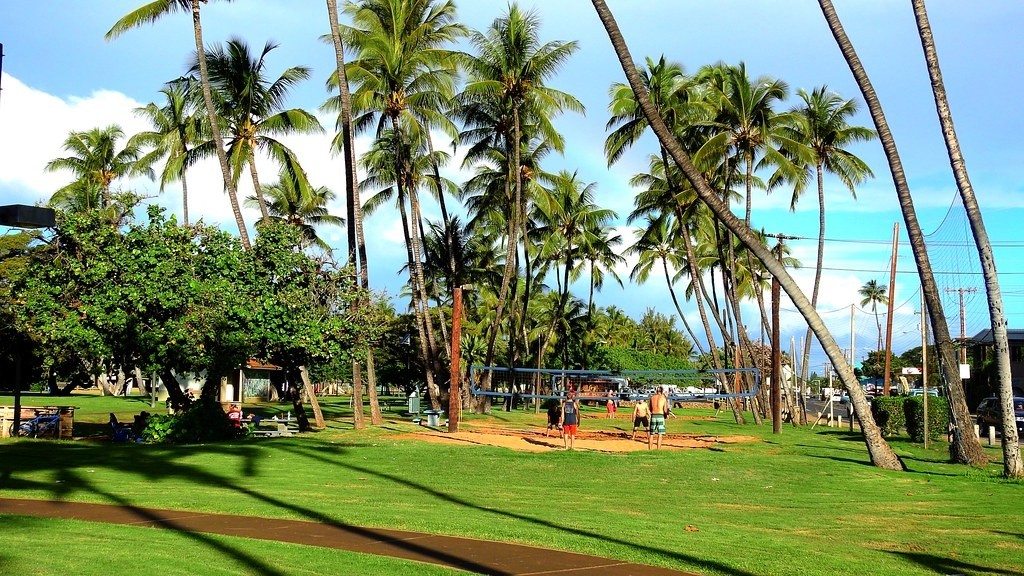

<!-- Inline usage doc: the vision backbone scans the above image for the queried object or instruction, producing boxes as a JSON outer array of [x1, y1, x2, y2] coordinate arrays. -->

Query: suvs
[[976, 396, 1024, 439]]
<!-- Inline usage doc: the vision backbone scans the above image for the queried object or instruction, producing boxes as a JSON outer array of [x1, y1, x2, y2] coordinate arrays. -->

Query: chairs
[[110, 413, 135, 443]]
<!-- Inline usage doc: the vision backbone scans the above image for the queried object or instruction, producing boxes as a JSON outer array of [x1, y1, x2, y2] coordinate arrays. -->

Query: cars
[[820, 384, 883, 406], [618, 380, 719, 397]]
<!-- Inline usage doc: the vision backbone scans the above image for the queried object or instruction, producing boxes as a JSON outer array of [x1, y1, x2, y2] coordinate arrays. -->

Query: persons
[[648, 387, 667, 450], [227, 404, 240, 431], [606, 389, 621, 419], [630, 398, 650, 438], [546, 393, 581, 449]]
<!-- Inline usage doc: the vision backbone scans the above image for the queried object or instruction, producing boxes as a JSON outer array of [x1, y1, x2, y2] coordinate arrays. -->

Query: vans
[[903, 389, 939, 397]]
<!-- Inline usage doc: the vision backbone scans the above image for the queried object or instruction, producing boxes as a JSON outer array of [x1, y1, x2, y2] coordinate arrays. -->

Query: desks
[[423, 409, 444, 426], [241, 418, 295, 422]]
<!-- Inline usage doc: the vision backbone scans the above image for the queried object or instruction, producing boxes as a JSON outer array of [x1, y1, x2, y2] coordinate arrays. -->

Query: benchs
[[445, 419, 449, 427], [252, 430, 300, 433], [413, 418, 422, 425]]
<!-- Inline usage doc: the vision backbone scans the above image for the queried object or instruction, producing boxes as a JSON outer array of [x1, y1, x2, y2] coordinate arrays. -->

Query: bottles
[[288, 411, 290, 420], [281, 413, 284, 420]]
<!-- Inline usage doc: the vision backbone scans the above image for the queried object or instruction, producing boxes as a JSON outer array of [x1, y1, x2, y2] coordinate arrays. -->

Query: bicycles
[[8, 405, 82, 439]]
